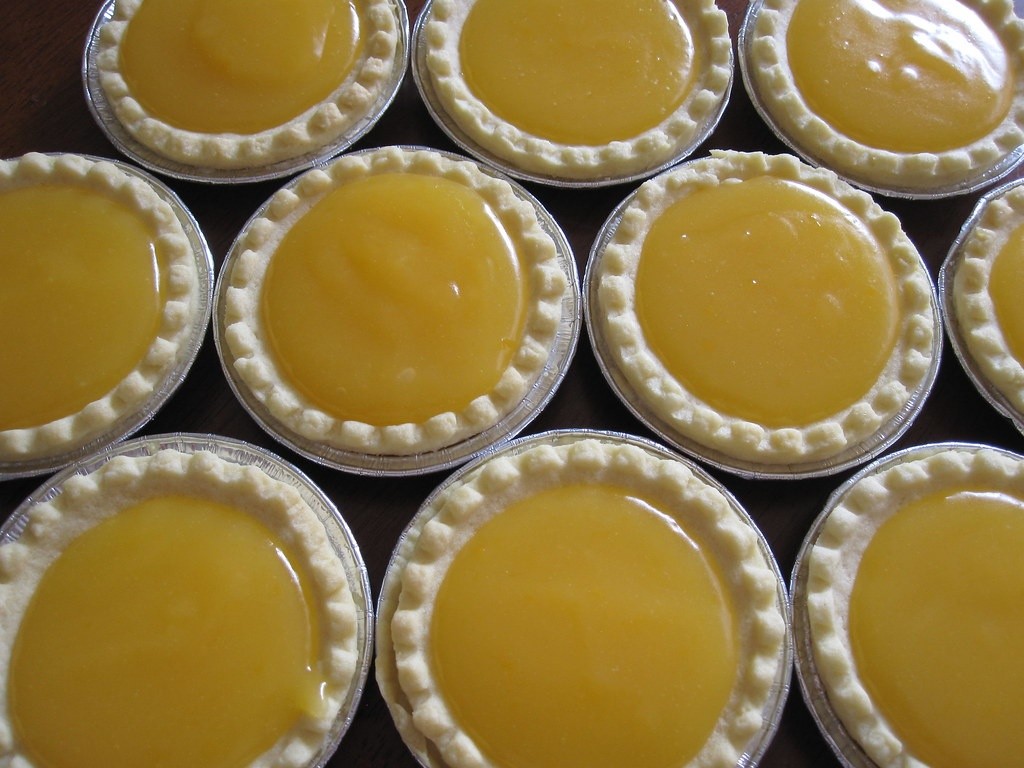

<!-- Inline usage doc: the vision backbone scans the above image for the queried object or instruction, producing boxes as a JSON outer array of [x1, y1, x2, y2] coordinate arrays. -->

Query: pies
[[0, 0, 1023, 768]]
